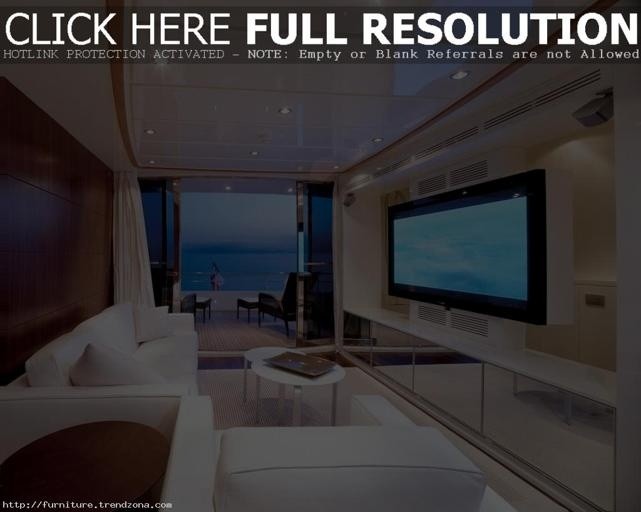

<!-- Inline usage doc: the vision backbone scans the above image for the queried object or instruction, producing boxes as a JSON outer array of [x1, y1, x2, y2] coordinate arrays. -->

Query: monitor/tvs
[[388, 168, 548, 326]]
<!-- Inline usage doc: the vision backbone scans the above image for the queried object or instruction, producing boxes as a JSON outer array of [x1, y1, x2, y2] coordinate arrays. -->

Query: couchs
[[1, 303, 200, 466]]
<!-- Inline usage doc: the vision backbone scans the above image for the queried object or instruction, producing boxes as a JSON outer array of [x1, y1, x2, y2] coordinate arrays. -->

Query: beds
[[237, 297, 264, 323]]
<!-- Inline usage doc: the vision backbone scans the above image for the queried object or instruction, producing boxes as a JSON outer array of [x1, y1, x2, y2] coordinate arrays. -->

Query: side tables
[[0, 421, 171, 511]]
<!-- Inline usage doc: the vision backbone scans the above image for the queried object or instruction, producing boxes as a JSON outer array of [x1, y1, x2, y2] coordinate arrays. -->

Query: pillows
[[71, 341, 166, 389], [135, 304, 171, 342]]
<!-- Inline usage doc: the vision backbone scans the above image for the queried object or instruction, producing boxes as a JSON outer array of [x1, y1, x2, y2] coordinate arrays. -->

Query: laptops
[[263, 349, 334, 376]]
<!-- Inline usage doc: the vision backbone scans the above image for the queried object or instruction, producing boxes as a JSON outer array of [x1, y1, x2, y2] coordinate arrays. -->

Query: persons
[[210, 262, 224, 291]]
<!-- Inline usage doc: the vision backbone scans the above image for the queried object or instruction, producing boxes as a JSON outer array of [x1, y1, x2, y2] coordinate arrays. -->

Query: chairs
[[257, 271, 299, 338], [159, 393, 518, 512]]
